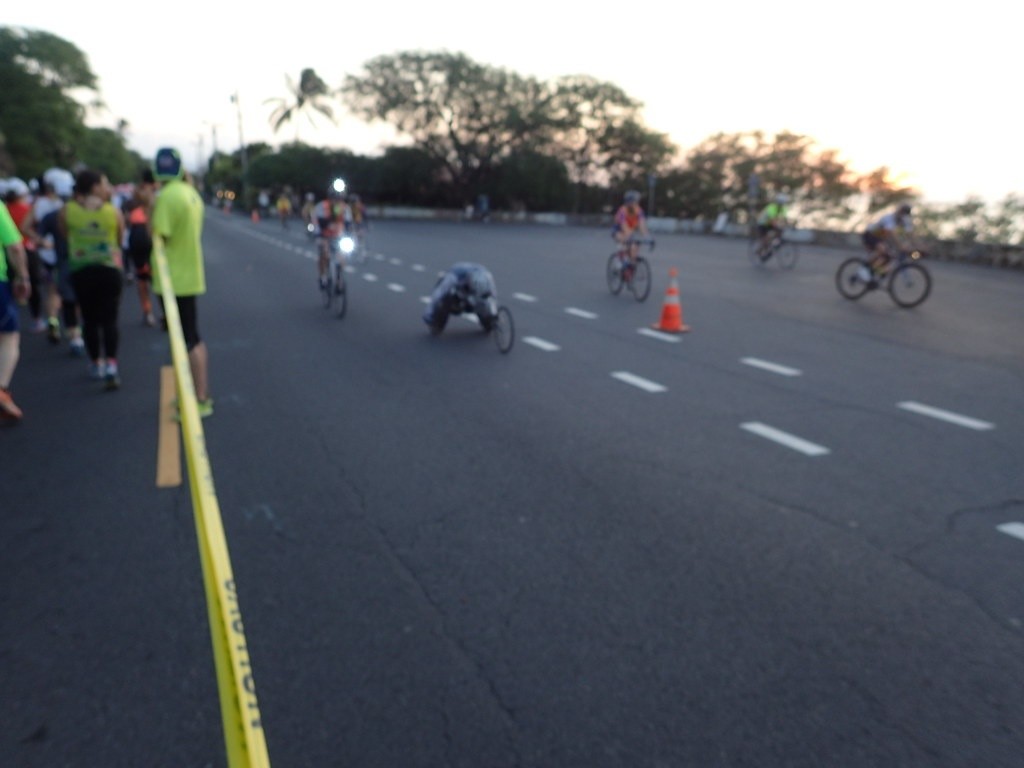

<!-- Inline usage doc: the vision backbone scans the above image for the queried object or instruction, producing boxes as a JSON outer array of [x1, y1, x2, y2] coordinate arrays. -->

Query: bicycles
[[833, 247, 932, 308], [304, 227, 348, 319], [606, 238, 655, 302], [746, 229, 797, 270]]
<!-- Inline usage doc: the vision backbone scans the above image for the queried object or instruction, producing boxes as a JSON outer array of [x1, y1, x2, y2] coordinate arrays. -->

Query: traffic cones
[[651, 268, 690, 332]]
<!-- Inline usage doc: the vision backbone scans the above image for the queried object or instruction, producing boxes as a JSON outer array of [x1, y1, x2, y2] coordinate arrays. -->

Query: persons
[[861, 203, 927, 288], [145, 147, 213, 424], [275, 178, 365, 292], [756, 192, 793, 257], [610, 189, 655, 281], [421, 262, 499, 330], [0, 165, 157, 417]]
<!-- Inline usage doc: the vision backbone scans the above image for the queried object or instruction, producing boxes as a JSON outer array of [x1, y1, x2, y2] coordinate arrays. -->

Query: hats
[[625, 190, 639, 200], [0, 177, 29, 196], [154, 147, 181, 181]]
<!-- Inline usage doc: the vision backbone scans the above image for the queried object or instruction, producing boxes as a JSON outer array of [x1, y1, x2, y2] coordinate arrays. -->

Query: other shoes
[[171, 399, 214, 421], [31, 318, 44, 334], [71, 339, 85, 352], [89, 365, 119, 390], [61, 326, 83, 340], [0, 388, 22, 419], [47, 317, 61, 343], [140, 309, 155, 328]]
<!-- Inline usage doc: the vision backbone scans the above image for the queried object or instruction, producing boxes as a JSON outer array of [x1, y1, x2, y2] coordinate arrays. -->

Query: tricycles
[[428, 270, 515, 354]]
